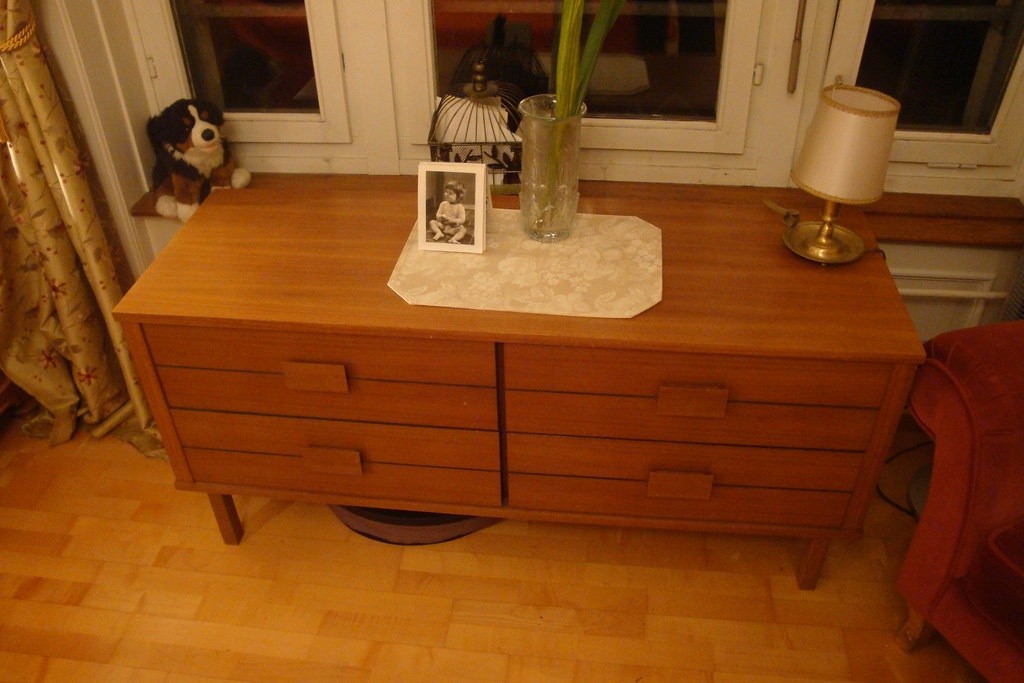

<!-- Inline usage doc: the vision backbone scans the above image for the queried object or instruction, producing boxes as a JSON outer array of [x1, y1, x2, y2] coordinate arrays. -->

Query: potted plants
[[518, 0, 631, 243]]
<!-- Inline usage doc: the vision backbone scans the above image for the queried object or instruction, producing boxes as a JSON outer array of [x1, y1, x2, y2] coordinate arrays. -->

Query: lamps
[[780, 85, 902, 265]]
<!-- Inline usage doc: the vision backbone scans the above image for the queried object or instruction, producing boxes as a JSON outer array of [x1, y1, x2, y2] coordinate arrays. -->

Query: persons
[[430, 180, 467, 244]]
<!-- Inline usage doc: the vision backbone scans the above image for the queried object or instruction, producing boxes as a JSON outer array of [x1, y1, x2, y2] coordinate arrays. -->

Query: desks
[[112, 169, 925, 591]]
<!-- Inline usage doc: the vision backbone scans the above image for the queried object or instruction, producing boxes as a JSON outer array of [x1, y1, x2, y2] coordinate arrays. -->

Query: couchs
[[897, 319, 1024, 683]]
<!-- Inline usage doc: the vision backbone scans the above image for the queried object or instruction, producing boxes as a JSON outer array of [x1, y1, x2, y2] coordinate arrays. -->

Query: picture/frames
[[418, 160, 488, 255]]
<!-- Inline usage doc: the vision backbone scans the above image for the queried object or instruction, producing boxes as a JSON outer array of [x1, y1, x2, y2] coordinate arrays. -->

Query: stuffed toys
[[146, 99, 251, 223]]
